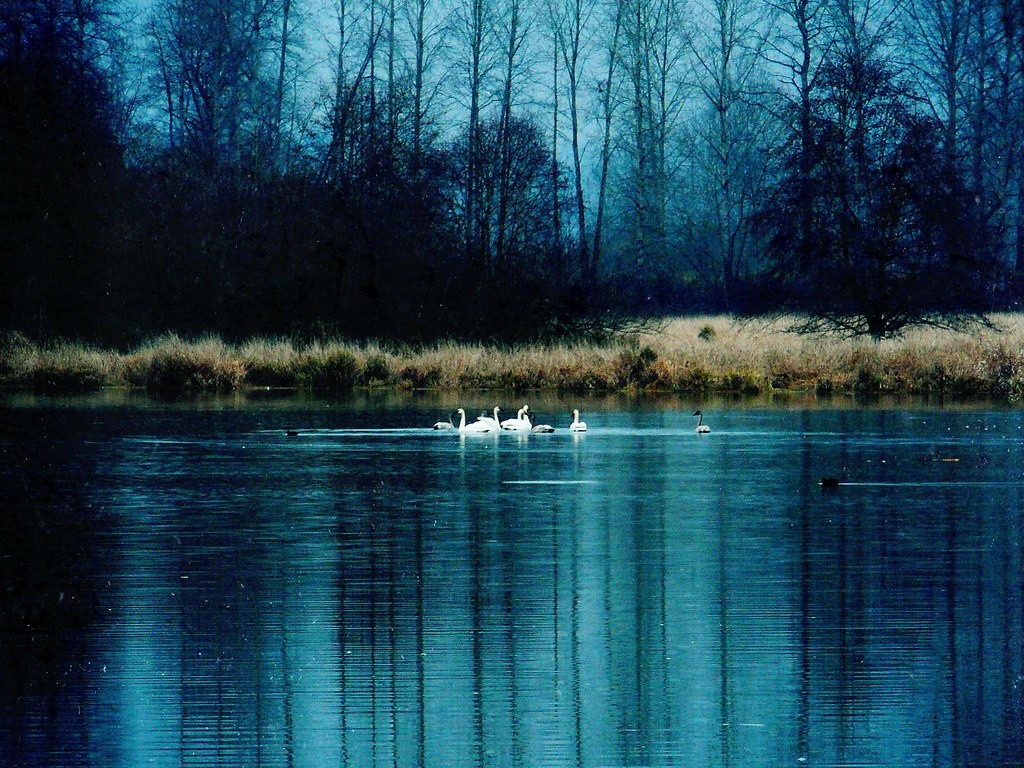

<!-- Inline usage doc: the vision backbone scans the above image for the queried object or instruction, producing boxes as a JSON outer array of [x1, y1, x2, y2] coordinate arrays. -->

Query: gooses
[[434, 405, 587, 433], [693, 411, 711, 433]]
